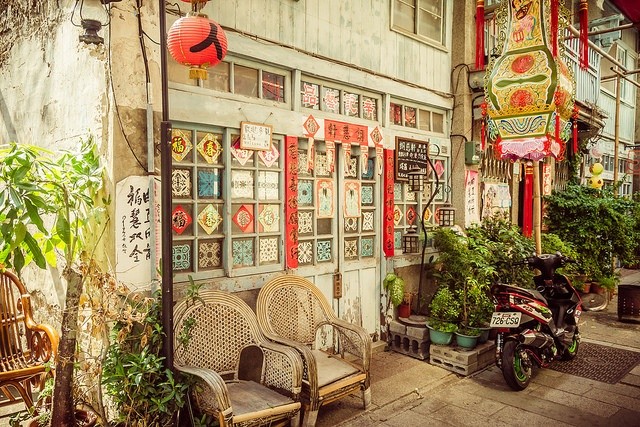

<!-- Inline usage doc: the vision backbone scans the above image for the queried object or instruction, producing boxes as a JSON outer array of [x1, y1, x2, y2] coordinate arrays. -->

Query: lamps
[[407, 161, 427, 192], [401, 220, 420, 254], [438, 199, 458, 228]]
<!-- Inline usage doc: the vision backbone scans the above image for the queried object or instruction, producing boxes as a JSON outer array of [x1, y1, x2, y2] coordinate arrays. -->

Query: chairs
[[256, 273, 375, 427], [0, 268, 61, 414], [172, 288, 304, 427]]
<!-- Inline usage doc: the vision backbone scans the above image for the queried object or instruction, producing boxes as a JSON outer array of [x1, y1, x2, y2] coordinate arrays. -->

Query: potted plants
[[419, 136, 640, 351], [0, 128, 112, 427], [398, 297, 410, 318]]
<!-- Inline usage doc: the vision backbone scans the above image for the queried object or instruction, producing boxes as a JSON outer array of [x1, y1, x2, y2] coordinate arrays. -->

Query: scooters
[[487, 250, 583, 392]]
